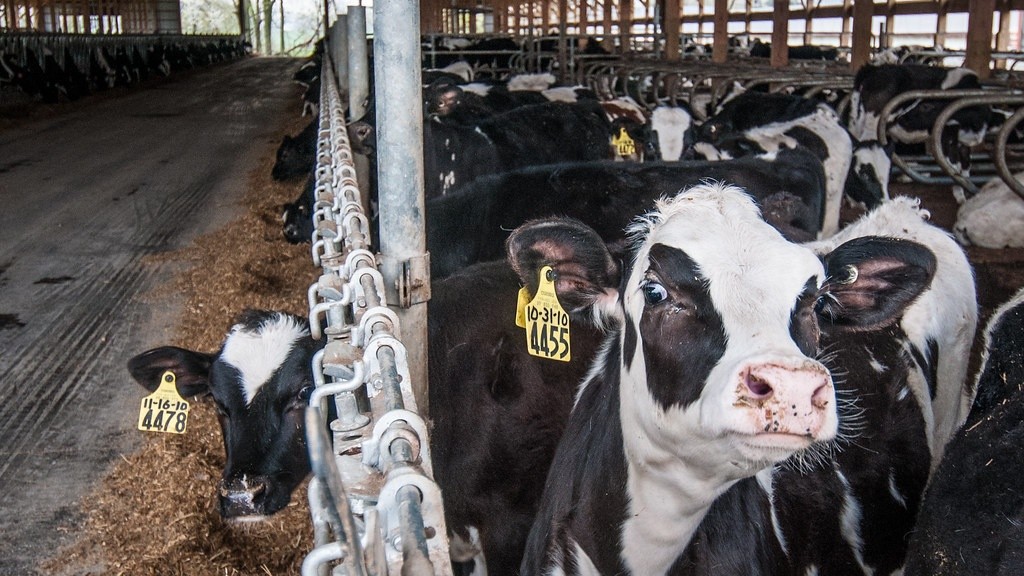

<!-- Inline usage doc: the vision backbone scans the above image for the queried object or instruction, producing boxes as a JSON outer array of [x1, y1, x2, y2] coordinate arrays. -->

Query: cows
[[124, 33, 1024, 576], [0, 38, 253, 105]]
